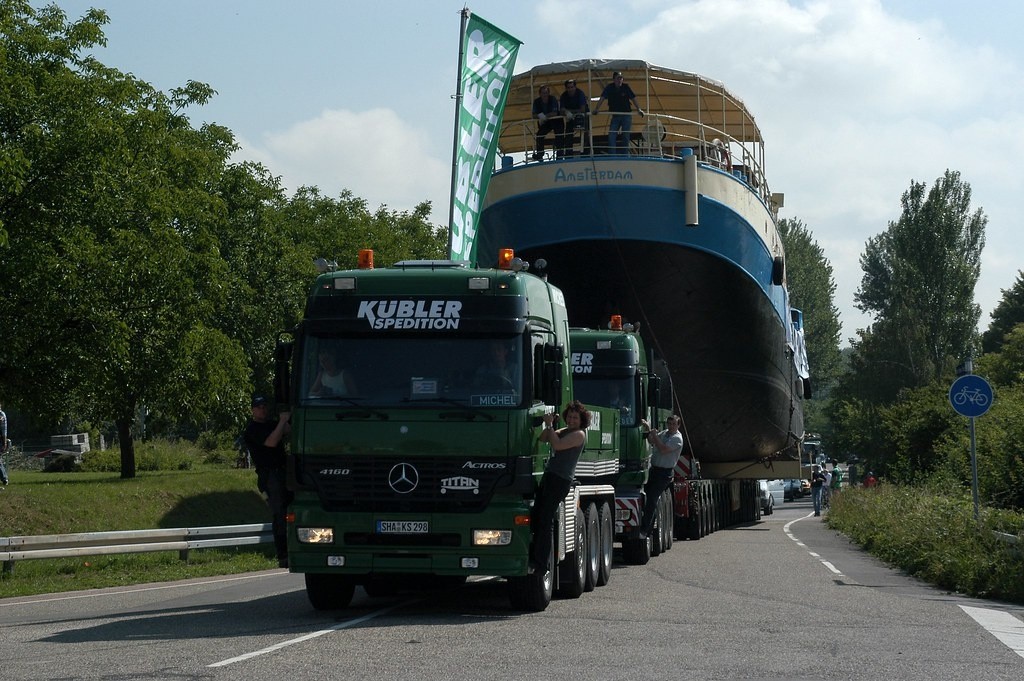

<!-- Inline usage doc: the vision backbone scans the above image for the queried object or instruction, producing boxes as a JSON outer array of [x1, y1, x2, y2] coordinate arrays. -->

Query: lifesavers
[[772, 256, 784, 285], [711, 138, 730, 170]]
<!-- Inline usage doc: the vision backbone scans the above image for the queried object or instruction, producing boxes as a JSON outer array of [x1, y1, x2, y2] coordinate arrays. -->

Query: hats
[[613, 71, 623, 78], [251, 395, 267, 409]]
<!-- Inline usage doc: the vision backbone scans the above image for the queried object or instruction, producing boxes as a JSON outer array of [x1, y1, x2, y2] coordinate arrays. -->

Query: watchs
[[547, 425, 553, 430]]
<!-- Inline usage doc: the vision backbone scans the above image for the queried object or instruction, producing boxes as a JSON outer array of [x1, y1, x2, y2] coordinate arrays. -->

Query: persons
[[863, 472, 876, 488], [244, 394, 290, 569], [0, 404, 9, 486], [308, 344, 355, 396], [475, 341, 519, 395], [559, 79, 590, 158], [592, 71, 645, 154], [629, 414, 683, 542], [528, 399, 592, 573], [808, 458, 842, 517], [532, 85, 564, 162]]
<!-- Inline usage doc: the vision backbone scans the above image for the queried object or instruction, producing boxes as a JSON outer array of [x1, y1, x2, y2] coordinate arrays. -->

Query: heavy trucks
[[273, 247, 762, 614]]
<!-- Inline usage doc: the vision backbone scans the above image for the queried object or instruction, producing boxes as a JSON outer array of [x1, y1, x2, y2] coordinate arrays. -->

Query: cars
[[757, 464, 823, 515]]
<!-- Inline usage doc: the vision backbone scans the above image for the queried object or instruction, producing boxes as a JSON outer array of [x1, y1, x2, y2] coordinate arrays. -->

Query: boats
[[475, 59, 805, 464]]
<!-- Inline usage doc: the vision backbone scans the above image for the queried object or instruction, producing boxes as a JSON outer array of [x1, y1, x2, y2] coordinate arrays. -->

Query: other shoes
[[279, 556, 288, 567], [528, 558, 547, 577], [533, 154, 543, 162], [639, 529, 647, 535]]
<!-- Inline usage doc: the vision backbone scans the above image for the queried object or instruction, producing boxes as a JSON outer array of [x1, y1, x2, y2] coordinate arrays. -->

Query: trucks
[[802, 433, 826, 468]]
